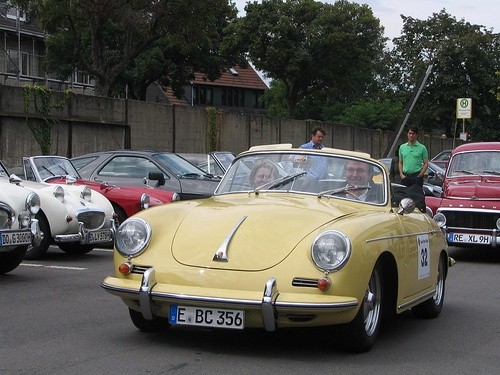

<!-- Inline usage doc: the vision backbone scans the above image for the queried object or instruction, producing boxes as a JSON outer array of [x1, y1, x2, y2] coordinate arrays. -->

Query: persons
[[398, 125, 429, 186], [249, 162, 280, 194], [332, 154, 382, 203], [295, 127, 329, 194]]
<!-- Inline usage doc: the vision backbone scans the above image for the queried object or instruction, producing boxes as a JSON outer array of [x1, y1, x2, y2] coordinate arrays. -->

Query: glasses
[[347, 167, 366, 173]]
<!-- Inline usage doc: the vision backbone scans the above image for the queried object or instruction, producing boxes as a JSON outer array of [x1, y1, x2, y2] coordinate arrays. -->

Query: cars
[[38, 150, 254, 200], [175, 149, 304, 186], [436, 142, 500, 247], [100, 143, 456, 355], [371, 149, 465, 218], [0, 159, 41, 274], [22, 156, 181, 249], [18, 179, 119, 261]]
